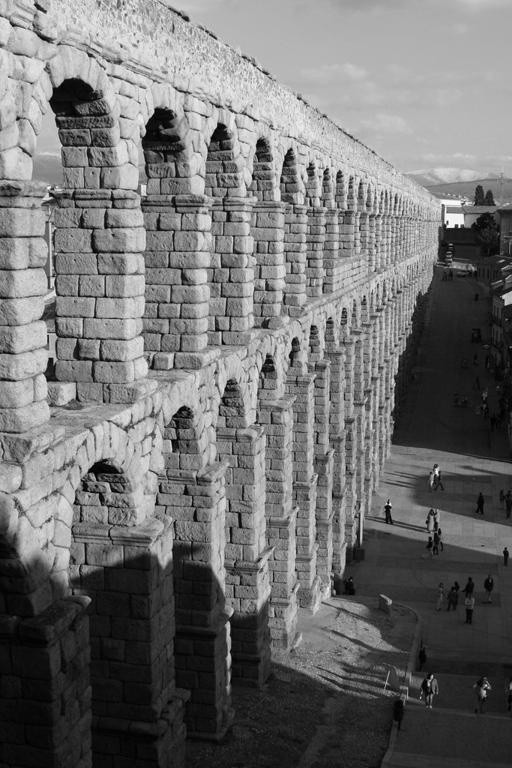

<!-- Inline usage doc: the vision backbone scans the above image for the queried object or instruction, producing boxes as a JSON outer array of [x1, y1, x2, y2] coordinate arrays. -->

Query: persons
[[435, 574, 493, 624], [500, 490, 512, 518], [476, 491, 484, 515], [473, 676, 512, 712], [425, 508, 443, 557], [419, 643, 439, 709], [429, 464, 446, 491], [384, 499, 393, 524], [503, 547, 509, 565], [344, 576, 355, 595]]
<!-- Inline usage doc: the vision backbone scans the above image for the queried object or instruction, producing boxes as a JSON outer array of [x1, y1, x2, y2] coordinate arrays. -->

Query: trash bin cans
[[355, 548, 364, 560]]
